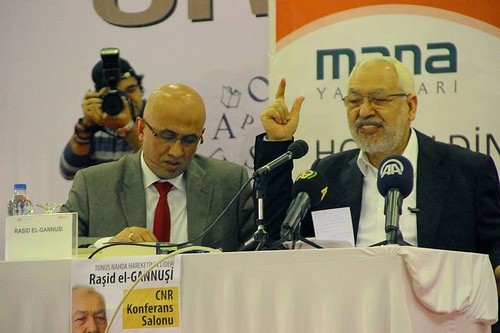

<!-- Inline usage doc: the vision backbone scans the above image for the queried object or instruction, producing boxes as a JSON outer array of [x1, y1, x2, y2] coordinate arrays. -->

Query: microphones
[[280, 168, 328, 239], [252, 140, 309, 178], [377, 155, 414, 245]]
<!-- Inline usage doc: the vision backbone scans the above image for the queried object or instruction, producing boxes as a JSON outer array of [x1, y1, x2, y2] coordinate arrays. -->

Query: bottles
[[7, 184, 33, 217]]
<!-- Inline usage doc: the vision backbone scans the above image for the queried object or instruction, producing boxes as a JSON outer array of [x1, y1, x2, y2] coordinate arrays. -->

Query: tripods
[[369, 230, 413, 247], [237, 177, 287, 252], [271, 221, 323, 250]]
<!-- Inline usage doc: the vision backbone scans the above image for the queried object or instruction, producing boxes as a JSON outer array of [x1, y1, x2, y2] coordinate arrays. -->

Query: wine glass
[[36, 202, 62, 214]]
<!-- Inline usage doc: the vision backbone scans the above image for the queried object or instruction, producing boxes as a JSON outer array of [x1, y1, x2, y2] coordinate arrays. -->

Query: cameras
[[100, 48, 132, 115]]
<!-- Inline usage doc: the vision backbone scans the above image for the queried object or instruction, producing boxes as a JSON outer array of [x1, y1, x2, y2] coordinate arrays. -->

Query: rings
[[128, 233, 134, 238]]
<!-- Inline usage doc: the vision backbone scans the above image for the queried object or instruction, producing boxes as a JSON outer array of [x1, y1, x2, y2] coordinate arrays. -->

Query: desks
[[0, 244, 498, 333]]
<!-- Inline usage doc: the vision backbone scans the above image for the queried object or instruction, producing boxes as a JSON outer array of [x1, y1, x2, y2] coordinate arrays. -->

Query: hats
[[92, 58, 134, 88]]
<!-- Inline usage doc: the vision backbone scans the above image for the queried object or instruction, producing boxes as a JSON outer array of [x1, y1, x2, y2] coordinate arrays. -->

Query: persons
[[71, 284, 110, 333], [57, 83, 257, 253], [254, 56, 497, 272], [58, 56, 147, 181]]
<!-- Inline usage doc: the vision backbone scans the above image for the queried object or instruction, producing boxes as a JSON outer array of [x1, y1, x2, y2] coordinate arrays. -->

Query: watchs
[[116, 119, 135, 137]]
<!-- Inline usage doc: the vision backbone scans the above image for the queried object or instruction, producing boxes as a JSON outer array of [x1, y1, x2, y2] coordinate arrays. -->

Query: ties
[[152, 182, 173, 242]]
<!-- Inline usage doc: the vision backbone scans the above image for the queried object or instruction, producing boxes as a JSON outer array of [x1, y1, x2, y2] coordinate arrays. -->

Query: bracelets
[[75, 134, 93, 144], [74, 119, 96, 133]]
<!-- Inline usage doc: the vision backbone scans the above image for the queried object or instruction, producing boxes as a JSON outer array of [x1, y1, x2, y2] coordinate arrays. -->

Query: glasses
[[143, 119, 203, 149], [341, 93, 411, 109]]
